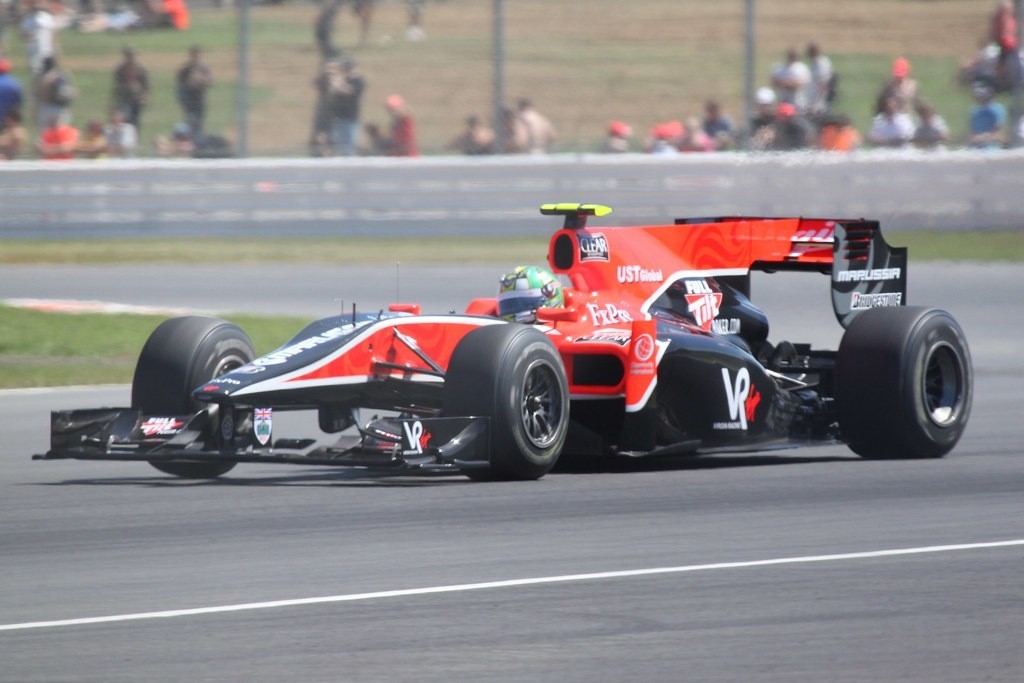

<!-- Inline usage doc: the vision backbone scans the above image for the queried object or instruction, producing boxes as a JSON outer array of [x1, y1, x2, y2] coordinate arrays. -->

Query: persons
[[745, 86, 781, 139], [777, 48, 812, 108], [966, 78, 1009, 151], [706, 98, 739, 145], [0, 1, 713, 155], [809, 44, 840, 117], [990, 3, 1024, 91], [494, 265, 566, 329], [911, 96, 951, 151], [819, 115, 862, 151], [880, 58, 921, 113], [776, 105, 822, 151], [768, 50, 795, 107], [867, 96, 917, 149]]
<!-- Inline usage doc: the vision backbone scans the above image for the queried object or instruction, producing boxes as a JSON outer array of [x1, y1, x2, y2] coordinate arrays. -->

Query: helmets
[[497, 265, 565, 326]]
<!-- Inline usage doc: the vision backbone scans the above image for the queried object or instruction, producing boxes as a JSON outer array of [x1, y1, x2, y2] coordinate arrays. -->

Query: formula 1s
[[24, 203, 976, 482]]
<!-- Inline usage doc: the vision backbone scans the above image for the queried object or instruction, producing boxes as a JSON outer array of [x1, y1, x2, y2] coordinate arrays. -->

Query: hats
[[891, 57, 910, 78], [386, 95, 405, 111], [610, 121, 631, 136], [757, 88, 776, 103], [999, 16, 1016, 35], [996, 7, 1016, 22], [999, 32, 1018, 49], [0, 59, 13, 72], [651, 124, 674, 140], [667, 120, 684, 137], [778, 103, 796, 118]]
